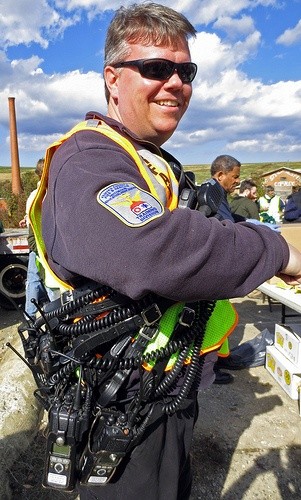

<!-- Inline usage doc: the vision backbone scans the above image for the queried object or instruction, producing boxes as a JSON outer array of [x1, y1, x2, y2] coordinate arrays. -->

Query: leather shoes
[[214, 354, 246, 370], [211, 367, 234, 384]]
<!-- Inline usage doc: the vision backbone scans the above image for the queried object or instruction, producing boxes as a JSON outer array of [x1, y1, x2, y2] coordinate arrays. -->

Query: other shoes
[[18, 320, 34, 332]]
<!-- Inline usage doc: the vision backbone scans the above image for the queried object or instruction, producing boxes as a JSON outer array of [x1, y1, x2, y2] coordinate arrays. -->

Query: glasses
[[112, 58, 197, 84]]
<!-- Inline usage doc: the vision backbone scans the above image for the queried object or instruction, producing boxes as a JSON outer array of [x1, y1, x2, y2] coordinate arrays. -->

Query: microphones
[[197, 178, 223, 215]]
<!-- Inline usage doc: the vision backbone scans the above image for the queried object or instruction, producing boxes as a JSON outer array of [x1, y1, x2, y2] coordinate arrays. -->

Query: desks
[[255, 281, 301, 324]]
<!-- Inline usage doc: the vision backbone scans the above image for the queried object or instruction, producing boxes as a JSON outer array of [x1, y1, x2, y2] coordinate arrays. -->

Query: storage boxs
[[0, 228, 36, 255], [274, 323, 301, 368], [263, 345, 301, 400]]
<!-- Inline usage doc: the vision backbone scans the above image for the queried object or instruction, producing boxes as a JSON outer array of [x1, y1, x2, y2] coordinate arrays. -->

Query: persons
[[19, 4, 301, 500]]
